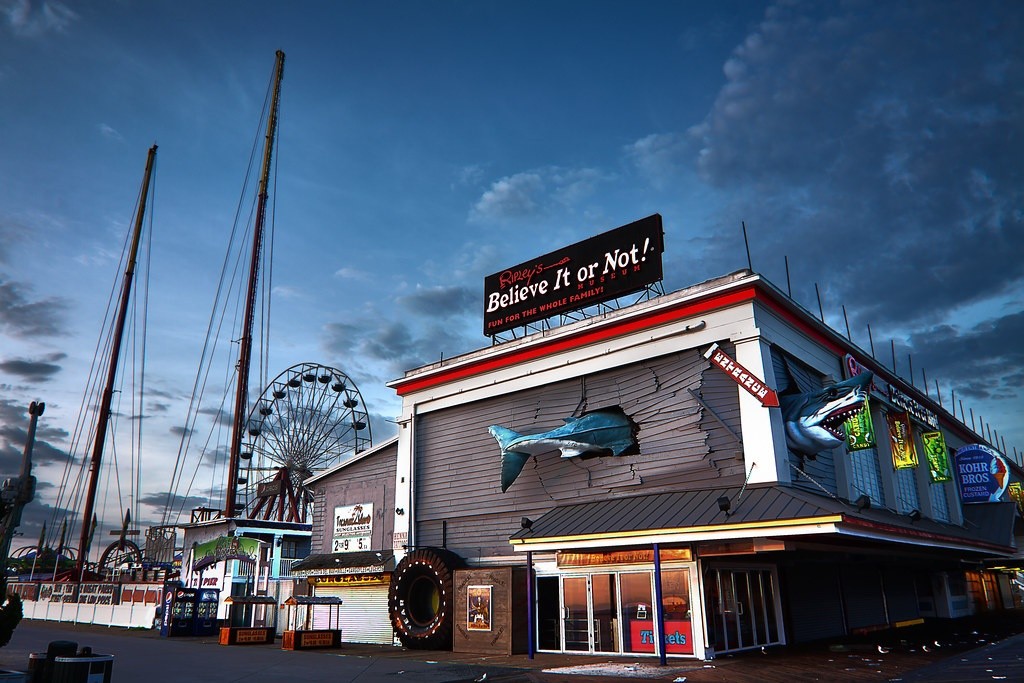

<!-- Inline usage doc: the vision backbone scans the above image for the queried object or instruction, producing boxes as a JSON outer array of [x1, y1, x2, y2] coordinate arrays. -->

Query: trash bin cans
[[27, 640, 115, 683]]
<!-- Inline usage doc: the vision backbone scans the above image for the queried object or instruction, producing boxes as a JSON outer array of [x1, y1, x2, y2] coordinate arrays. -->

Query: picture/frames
[[467, 585, 493, 632]]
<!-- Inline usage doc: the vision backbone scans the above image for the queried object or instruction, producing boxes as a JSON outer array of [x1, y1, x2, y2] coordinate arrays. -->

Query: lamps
[[396, 508, 404, 516], [717, 497, 732, 516], [521, 517, 536, 534], [855, 496, 871, 514], [906, 511, 921, 525]]
